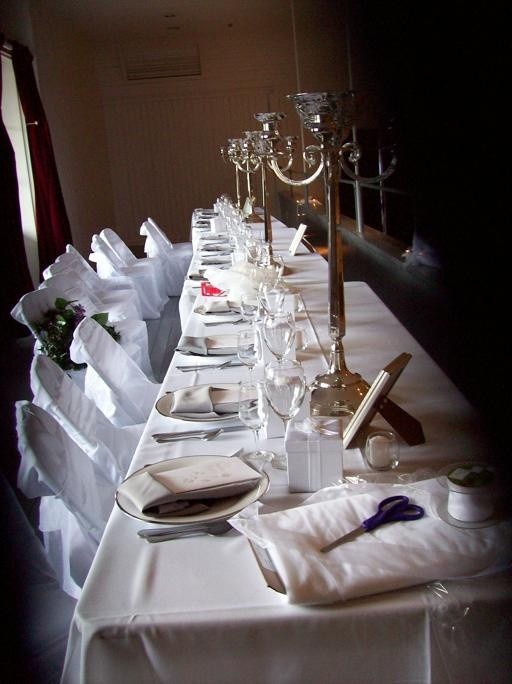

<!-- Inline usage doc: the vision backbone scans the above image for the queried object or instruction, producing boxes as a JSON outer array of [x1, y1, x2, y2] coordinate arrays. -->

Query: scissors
[[320, 496, 425, 552]]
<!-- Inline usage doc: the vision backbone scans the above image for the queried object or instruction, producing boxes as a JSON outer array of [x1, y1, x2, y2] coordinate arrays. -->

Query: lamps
[[218, 92, 404, 438]]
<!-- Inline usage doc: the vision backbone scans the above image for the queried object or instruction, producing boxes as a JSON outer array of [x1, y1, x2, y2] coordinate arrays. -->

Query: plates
[[114, 454, 271, 526], [156, 383, 258, 422], [180, 333, 243, 355]]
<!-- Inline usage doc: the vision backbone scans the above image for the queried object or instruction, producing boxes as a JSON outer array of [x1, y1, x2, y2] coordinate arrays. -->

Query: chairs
[[0, 216, 192, 683]]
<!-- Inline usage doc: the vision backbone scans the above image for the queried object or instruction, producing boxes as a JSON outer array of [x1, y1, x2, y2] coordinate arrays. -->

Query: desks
[[58, 206, 512, 683]]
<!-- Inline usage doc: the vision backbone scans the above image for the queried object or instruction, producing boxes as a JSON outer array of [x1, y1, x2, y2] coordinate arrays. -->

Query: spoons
[[147, 522, 233, 543], [182, 361, 231, 372], [205, 318, 243, 327], [156, 429, 223, 443]]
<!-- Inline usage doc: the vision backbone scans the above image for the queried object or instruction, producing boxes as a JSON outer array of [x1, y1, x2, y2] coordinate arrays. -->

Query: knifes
[[152, 424, 261, 439]]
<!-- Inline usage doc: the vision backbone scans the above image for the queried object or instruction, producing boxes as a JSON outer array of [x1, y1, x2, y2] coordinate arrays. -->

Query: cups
[[366, 431, 399, 471]]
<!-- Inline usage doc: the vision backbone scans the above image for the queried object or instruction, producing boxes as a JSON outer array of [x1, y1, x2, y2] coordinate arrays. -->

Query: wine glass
[[238, 380, 277, 464], [214, 194, 285, 325], [237, 327, 262, 387], [263, 359, 306, 470], [264, 310, 296, 377]]
